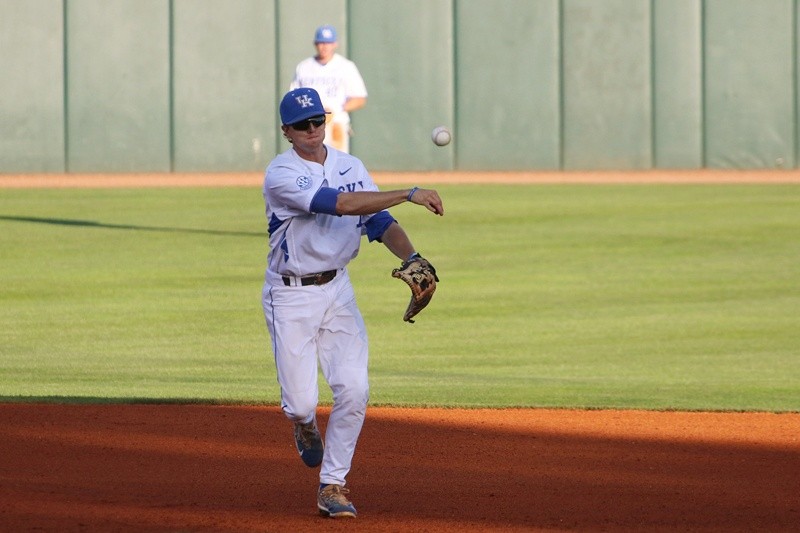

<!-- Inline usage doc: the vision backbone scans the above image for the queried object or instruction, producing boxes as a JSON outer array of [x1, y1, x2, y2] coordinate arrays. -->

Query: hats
[[280, 88, 332, 126], [313, 25, 336, 43]]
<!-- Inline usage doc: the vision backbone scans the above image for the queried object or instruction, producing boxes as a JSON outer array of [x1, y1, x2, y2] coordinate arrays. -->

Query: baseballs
[[432, 126, 451, 147]]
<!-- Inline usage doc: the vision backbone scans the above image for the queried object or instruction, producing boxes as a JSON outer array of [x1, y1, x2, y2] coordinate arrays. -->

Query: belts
[[280, 269, 337, 286]]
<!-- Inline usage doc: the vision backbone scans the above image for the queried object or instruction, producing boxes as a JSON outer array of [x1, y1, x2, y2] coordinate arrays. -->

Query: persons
[[288, 26, 368, 154], [261, 87, 446, 517]]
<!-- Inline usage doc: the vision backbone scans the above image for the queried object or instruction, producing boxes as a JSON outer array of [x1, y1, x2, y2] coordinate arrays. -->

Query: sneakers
[[293, 417, 324, 469], [317, 482, 358, 518]]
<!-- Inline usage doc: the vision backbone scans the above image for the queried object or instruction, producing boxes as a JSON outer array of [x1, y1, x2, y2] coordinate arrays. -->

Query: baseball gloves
[[391, 258, 438, 323]]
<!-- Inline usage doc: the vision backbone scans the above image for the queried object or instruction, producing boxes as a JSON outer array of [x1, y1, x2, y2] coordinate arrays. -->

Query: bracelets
[[407, 186, 419, 201]]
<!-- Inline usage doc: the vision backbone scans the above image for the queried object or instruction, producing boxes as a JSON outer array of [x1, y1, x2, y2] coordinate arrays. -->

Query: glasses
[[293, 115, 326, 130]]
